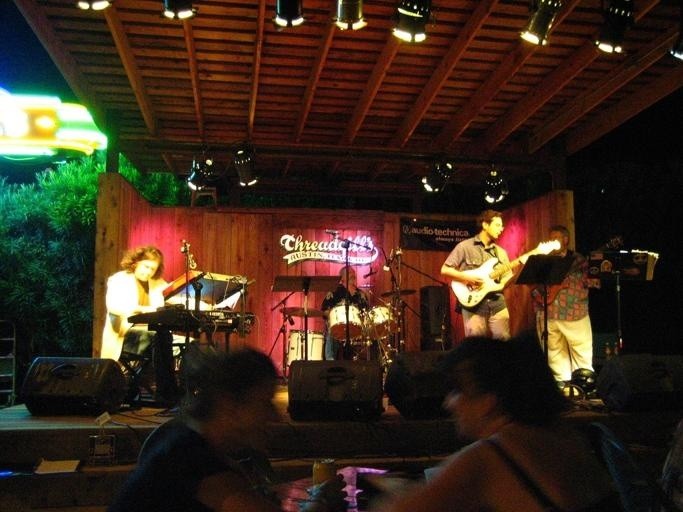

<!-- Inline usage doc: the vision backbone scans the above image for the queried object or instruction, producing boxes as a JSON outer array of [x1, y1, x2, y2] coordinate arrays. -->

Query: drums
[[328, 305, 363, 338], [363, 304, 397, 340], [287, 329, 326, 371]]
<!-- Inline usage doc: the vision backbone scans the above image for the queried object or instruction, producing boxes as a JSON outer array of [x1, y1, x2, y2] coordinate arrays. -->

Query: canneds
[[312, 455, 337, 486]]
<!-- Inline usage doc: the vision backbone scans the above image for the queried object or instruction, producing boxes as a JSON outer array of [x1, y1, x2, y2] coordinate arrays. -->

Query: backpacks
[[581, 416, 682, 512]]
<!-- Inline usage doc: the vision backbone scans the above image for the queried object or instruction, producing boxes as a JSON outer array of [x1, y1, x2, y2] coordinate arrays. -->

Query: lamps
[[669, 33, 683, 60], [594, 0, 633, 54], [484, 163, 508, 204], [187, 152, 215, 191], [232, 148, 260, 186], [389, 1, 433, 44], [271, 1, 306, 28], [520, 0, 561, 45], [333, 1, 368, 31], [160, 1, 198, 19], [421, 158, 456, 193], [75, 1, 113, 12]]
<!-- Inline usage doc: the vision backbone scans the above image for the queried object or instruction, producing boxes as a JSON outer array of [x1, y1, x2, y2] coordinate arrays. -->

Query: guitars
[[451, 239, 561, 307], [531, 236, 623, 305]]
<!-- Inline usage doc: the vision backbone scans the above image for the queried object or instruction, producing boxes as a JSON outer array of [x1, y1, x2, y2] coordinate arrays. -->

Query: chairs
[[595, 424, 679, 512]]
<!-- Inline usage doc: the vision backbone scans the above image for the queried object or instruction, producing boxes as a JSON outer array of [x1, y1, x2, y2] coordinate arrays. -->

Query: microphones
[[188, 254, 197, 269], [363, 272, 377, 278], [325, 229, 338, 234], [383, 259, 391, 272]]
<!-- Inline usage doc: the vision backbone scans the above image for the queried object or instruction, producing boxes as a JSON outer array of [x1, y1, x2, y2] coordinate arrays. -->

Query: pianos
[[128, 309, 256, 332]]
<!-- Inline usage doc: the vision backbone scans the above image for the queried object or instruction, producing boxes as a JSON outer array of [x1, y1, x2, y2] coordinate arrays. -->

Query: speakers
[[20, 356, 127, 417], [385, 350, 453, 420], [288, 359, 383, 422], [420, 285, 451, 337]]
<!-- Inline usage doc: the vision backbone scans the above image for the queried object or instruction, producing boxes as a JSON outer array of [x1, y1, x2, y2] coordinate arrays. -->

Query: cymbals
[[279, 307, 321, 317], [382, 289, 416, 297]]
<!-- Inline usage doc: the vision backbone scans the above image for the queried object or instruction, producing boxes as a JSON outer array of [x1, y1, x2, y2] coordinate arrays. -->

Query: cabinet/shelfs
[[0, 320, 17, 406]]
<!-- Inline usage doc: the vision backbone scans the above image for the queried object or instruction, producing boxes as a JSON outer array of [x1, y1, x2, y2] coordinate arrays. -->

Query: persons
[[101, 246, 173, 407], [529, 224, 600, 396], [103, 343, 281, 512], [441, 211, 524, 341], [322, 265, 368, 360], [382, 335, 580, 512]]
